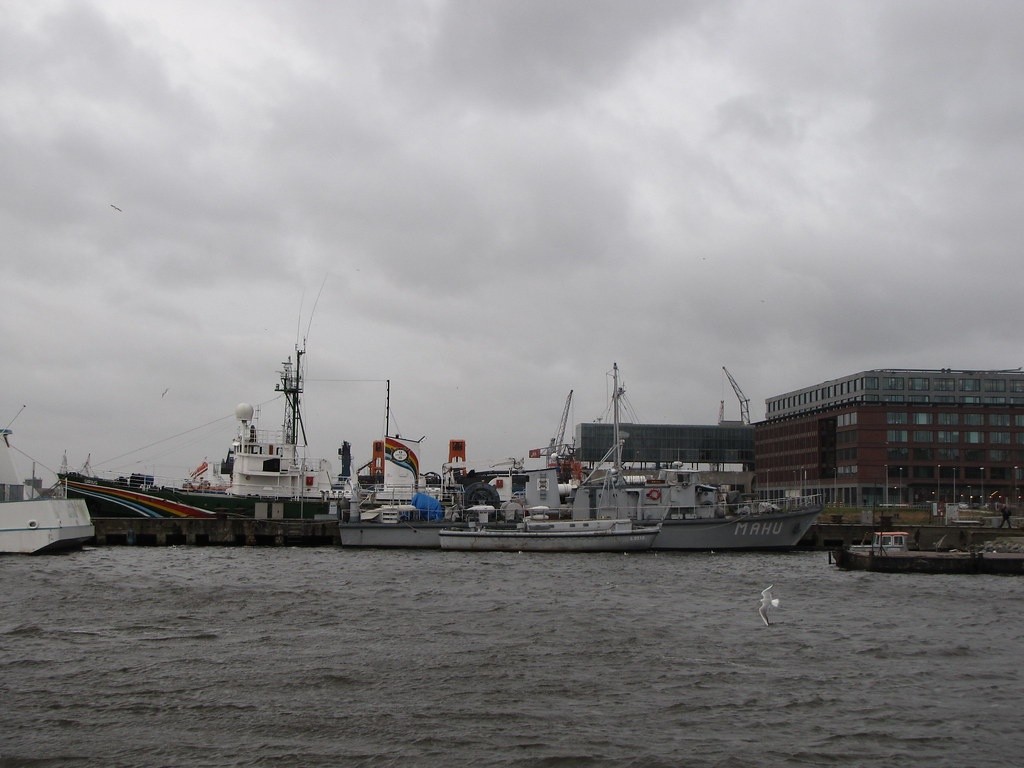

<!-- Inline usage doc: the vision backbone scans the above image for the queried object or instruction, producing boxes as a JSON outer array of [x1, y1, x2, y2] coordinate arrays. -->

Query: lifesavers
[[648, 488, 662, 500]]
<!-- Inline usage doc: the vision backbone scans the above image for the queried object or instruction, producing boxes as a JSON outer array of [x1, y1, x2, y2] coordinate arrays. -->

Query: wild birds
[[161, 388, 169, 397], [111, 204, 122, 212], [758, 584, 780, 627]]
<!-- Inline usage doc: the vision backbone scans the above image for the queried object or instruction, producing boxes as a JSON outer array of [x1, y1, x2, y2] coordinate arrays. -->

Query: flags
[[190, 457, 208, 480]]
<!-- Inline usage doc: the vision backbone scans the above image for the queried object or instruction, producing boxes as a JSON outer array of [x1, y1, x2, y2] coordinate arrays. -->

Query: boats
[[822, 532, 1024, 572], [58, 339, 578, 520], [0, 404, 140, 555], [438, 519, 661, 551], [337, 365, 824, 548]]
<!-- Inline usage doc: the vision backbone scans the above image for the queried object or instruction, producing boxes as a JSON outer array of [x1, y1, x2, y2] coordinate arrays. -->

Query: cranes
[[721, 367, 751, 426], [555, 390, 574, 458]]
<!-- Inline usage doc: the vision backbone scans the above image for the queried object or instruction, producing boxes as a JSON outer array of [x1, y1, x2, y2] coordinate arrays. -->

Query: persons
[[998, 504, 1012, 529]]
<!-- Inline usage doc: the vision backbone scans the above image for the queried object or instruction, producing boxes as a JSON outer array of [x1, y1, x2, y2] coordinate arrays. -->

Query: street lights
[[767, 469, 770, 503], [899, 467, 902, 505], [979, 467, 984, 508], [884, 464, 888, 506], [953, 467, 956, 504], [937, 464, 941, 503], [832, 467, 837, 503], [800, 466, 804, 505]]
